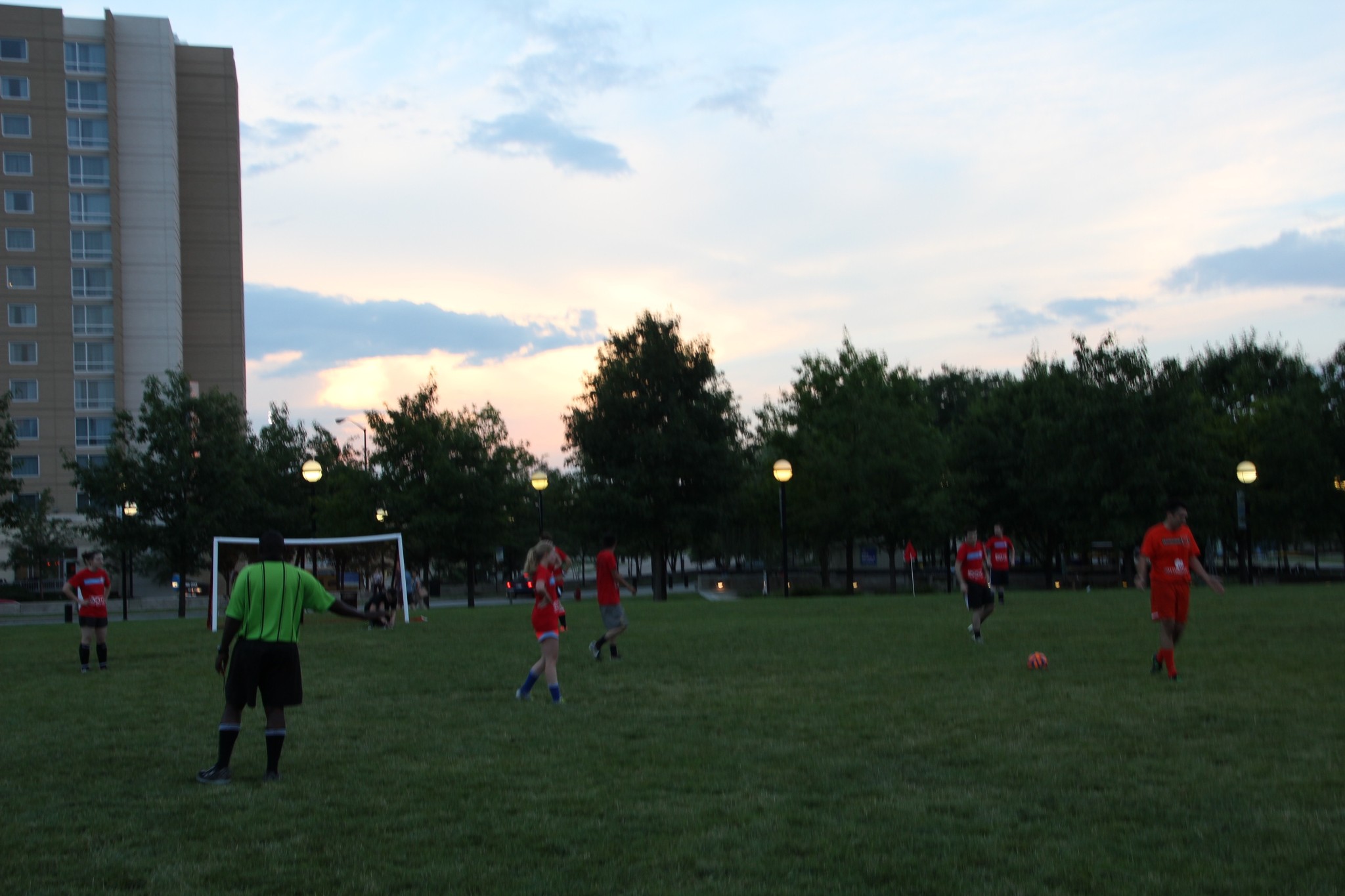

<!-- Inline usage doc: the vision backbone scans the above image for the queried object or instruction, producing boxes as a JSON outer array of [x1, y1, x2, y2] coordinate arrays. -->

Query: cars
[[506, 572, 536, 597]]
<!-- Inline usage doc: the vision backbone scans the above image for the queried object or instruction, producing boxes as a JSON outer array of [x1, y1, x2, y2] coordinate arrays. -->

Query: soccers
[[1027, 652, 1047, 669]]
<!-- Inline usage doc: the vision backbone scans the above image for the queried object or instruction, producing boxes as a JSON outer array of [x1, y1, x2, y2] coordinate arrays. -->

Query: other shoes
[[557, 696, 567, 705], [1150, 654, 1163, 675], [560, 626, 567, 631], [81, 667, 90, 673], [588, 640, 602, 661], [516, 688, 532, 701]]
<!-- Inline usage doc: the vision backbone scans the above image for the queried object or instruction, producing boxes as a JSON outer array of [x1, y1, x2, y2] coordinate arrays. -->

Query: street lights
[[302, 460, 323, 578], [375, 508, 388, 587], [773, 458, 792, 596], [1235, 459, 1258, 584], [531, 471, 548, 540], [124, 500, 138, 598]]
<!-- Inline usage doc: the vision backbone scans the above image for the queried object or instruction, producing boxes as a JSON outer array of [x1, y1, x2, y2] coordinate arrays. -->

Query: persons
[[362, 564, 428, 628], [984, 521, 1016, 607], [62, 549, 111, 672], [588, 535, 636, 661], [954, 525, 995, 642], [1134, 502, 1225, 682], [514, 529, 570, 705], [196, 529, 392, 785]]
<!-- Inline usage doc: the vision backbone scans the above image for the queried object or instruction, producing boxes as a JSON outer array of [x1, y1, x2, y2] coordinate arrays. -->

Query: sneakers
[[264, 774, 280, 782], [197, 764, 232, 783]]
